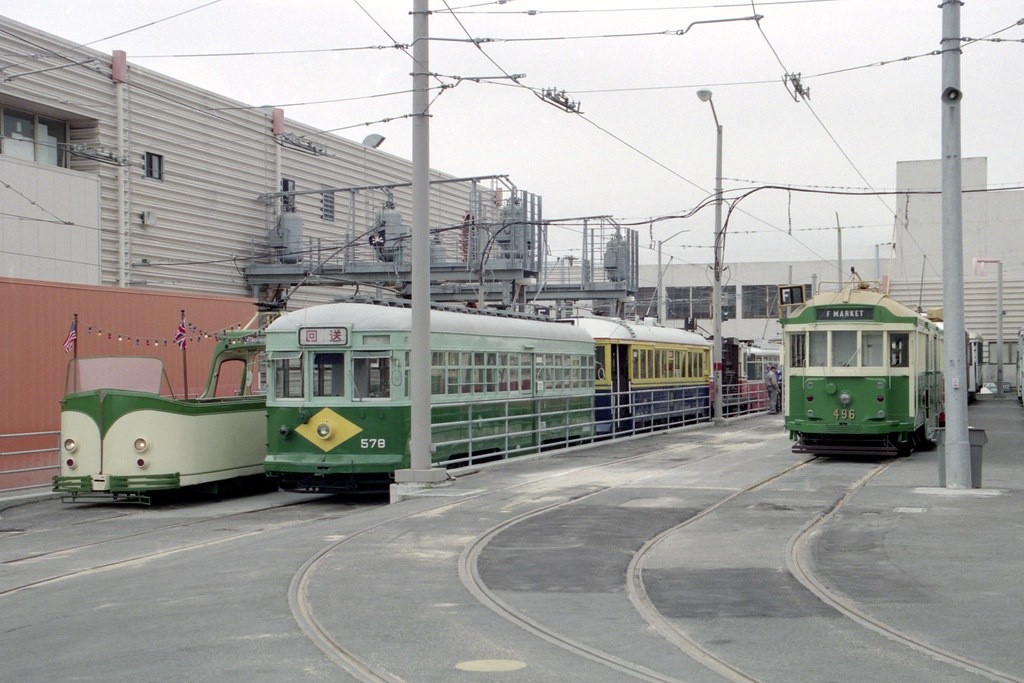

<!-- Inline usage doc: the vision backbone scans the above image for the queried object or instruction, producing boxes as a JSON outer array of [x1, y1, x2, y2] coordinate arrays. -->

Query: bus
[[554, 316, 712, 434], [263, 293, 598, 497], [52, 312, 267, 507], [1016, 330, 1024, 406], [707, 333, 784, 414], [776, 282, 985, 459]]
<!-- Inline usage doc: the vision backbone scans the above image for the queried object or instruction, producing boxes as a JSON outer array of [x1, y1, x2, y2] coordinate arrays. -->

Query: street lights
[[695, 88, 726, 426]]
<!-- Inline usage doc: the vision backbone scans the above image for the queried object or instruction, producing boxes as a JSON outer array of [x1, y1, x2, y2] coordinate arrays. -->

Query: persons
[[765, 367, 781, 414], [776, 365, 781, 412]]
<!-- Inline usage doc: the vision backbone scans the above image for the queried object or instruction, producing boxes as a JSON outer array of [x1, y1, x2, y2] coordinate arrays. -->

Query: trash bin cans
[[930, 427, 989, 489]]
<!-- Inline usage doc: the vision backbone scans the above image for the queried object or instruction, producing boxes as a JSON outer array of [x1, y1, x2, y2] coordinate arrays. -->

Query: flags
[[62, 321, 77, 354], [173, 318, 187, 351]]
